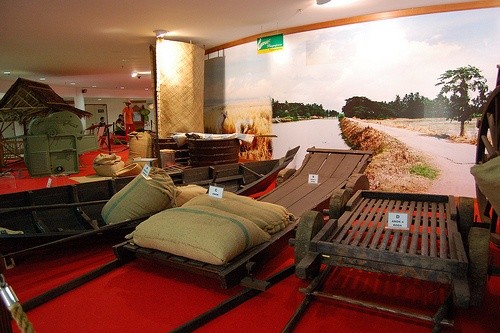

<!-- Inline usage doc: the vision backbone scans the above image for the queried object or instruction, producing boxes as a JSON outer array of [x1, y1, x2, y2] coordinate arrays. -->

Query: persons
[[119, 98, 137, 142], [98, 117, 107, 135], [116, 114, 124, 126]]
